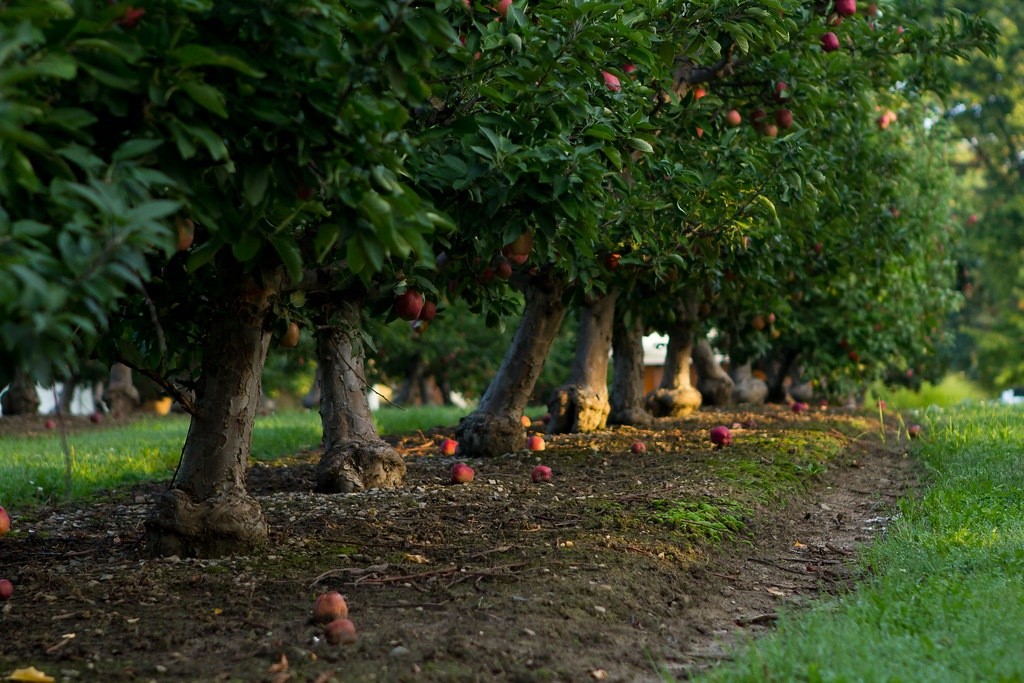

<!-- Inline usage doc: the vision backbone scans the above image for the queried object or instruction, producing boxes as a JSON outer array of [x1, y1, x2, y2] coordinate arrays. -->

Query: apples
[[45, 420, 55, 429], [0, 506, 11, 538], [90, 412, 103, 423], [311, 590, 357, 647], [175, 0, 926, 483], [0, 578, 14, 600]]
